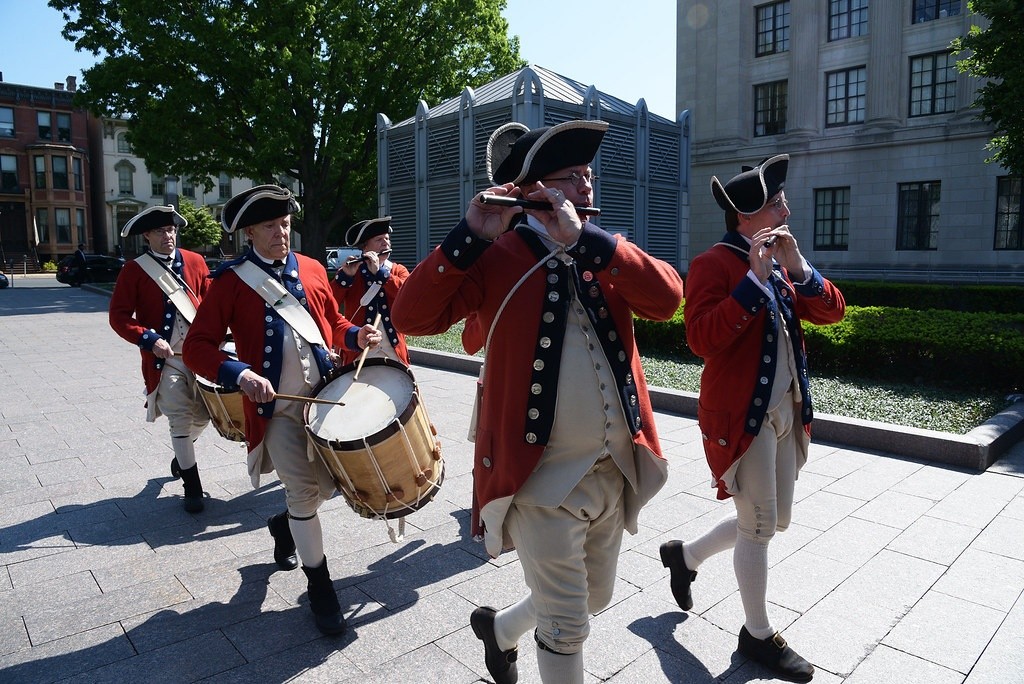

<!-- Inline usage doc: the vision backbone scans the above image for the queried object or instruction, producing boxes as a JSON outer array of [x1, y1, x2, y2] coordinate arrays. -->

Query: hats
[[346, 216, 392, 247], [709, 153, 790, 215], [221, 184, 301, 233], [486, 121, 610, 187], [121, 204, 188, 237]]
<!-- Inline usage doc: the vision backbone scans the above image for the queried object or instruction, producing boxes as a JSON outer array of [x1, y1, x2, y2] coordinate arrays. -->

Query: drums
[[191, 332, 248, 443], [303, 356, 446, 521]]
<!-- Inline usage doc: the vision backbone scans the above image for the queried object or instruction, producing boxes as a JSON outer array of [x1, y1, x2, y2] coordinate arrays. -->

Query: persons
[[389, 120, 683, 684], [183, 185, 384, 635], [74, 244, 123, 290], [330, 218, 411, 369], [659, 153, 846, 679], [110, 204, 214, 513]]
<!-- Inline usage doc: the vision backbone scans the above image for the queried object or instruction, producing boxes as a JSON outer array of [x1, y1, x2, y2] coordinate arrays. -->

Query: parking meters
[[9, 259, 14, 288], [23, 255, 27, 276]]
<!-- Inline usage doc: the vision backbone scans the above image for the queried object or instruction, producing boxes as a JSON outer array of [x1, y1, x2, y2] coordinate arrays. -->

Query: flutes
[[478, 193, 601, 215], [764, 233, 778, 249], [346, 248, 393, 266]]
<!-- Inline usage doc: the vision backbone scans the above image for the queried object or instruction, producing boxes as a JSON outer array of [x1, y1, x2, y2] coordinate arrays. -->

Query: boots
[[301, 555, 347, 634], [171, 438, 199, 479], [268, 509, 298, 570], [182, 462, 205, 514]]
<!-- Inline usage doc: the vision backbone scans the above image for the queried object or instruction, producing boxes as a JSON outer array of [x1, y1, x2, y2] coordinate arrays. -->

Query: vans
[[326, 247, 363, 270]]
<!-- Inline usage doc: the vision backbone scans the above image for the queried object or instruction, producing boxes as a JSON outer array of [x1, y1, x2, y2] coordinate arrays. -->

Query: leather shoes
[[660, 540, 698, 612], [470, 606, 518, 684], [738, 625, 815, 679]]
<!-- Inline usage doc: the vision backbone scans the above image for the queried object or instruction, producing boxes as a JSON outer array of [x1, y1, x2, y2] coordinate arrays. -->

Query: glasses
[[152, 228, 177, 236], [539, 173, 595, 186], [766, 198, 788, 210]]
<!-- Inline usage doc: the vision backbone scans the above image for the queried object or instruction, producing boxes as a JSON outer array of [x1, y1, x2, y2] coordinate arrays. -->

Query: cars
[[56, 254, 127, 287], [205, 257, 239, 277], [0, 274, 9, 288]]
